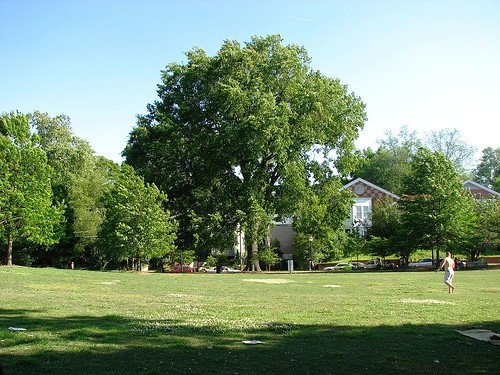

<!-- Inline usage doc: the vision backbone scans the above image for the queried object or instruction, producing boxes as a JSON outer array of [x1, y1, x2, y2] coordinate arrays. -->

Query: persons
[[434, 251, 456, 294]]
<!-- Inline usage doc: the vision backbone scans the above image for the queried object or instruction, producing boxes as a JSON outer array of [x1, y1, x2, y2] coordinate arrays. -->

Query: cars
[[323, 262, 353, 271], [351, 257, 465, 271], [171, 264, 240, 273]]
